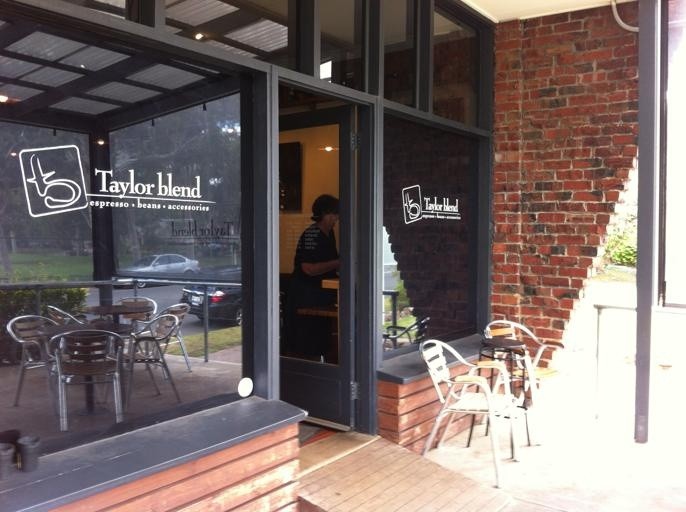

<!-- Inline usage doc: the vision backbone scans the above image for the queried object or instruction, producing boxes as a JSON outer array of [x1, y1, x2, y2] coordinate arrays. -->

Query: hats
[[313, 194, 340, 215]]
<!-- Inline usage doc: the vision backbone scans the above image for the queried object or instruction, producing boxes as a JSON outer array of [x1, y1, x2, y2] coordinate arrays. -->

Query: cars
[[110, 252, 201, 290], [179, 263, 245, 327]]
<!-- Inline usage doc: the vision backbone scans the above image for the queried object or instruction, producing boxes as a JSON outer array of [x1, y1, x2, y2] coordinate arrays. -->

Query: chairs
[[415, 316, 559, 490], [3, 292, 197, 436]]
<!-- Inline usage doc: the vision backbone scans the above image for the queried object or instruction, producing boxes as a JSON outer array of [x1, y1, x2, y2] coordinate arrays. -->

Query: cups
[[0, 442, 16, 479], [16, 435, 40, 471]]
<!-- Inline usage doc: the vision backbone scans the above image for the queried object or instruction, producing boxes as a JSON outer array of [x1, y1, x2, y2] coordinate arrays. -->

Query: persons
[[284, 193, 340, 363]]
